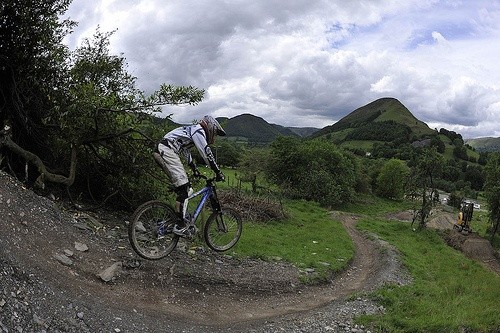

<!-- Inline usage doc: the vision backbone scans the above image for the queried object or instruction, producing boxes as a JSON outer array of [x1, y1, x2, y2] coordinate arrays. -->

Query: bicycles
[[128, 174, 243, 260]]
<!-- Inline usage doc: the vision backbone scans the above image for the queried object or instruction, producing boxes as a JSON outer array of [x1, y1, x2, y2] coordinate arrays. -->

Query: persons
[[152, 115, 226, 238]]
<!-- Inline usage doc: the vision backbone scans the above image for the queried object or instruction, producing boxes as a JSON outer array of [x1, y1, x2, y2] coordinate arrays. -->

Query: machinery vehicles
[[452, 201, 475, 235]]
